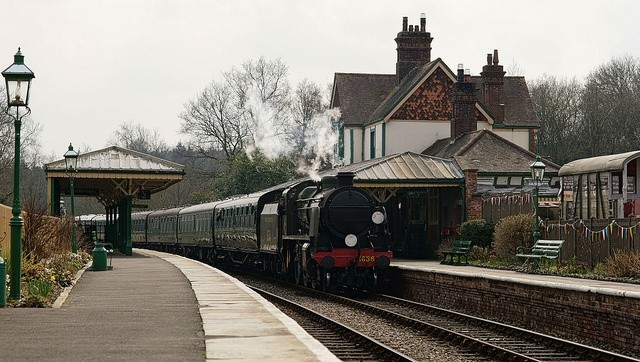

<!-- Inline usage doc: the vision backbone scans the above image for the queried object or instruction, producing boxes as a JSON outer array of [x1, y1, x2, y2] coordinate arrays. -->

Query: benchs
[[516, 239, 566, 265], [440, 240, 473, 265]]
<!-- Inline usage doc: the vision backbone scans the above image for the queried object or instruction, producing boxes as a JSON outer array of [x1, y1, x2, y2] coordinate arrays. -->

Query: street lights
[[63, 142, 79, 254], [60, 198, 65, 221], [1, 47, 35, 302], [529, 155, 547, 271]]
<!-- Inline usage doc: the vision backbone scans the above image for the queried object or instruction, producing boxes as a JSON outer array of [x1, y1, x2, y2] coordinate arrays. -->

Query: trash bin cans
[[0, 248, 7, 308], [93, 243, 107, 271]]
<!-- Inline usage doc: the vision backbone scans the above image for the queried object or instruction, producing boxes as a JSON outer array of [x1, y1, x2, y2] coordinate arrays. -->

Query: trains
[[70, 172, 394, 293]]
